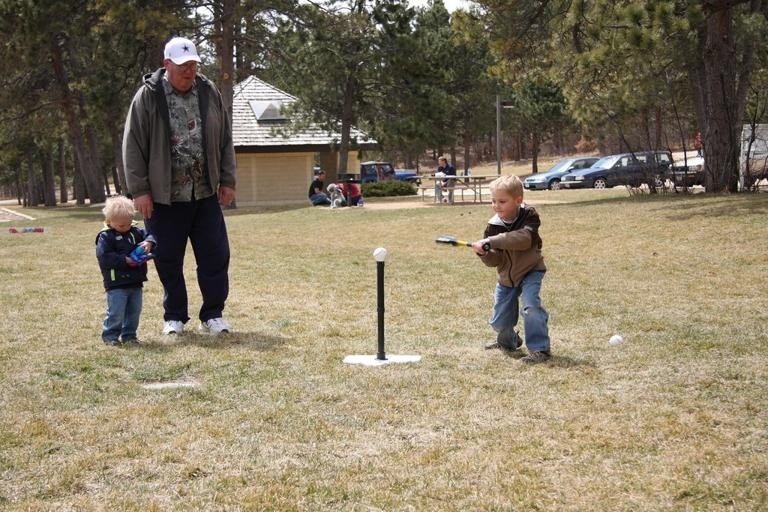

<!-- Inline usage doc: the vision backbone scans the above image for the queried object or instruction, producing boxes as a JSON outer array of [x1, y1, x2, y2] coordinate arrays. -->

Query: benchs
[[404, 169, 506, 203]]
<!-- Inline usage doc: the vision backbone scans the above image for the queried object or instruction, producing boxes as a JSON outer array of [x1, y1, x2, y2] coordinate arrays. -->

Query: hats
[[164, 37, 202, 65]]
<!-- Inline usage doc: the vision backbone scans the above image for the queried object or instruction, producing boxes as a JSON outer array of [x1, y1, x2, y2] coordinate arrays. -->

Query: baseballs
[[609, 335, 623, 347], [373, 248, 387, 261]]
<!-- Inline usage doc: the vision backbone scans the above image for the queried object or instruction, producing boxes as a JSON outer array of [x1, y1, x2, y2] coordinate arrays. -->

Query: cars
[[667, 122, 767, 192], [313, 166, 322, 181], [522, 155, 601, 191]]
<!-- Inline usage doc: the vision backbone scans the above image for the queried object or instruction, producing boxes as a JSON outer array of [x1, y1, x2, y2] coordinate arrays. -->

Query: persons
[[122, 37, 236, 334], [338, 183, 361, 206], [472, 175, 551, 364], [308, 171, 331, 206], [95, 196, 158, 346], [434, 157, 456, 205]]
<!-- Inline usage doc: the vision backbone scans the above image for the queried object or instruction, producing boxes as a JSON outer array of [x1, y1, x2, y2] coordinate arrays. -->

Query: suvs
[[359, 161, 421, 192]]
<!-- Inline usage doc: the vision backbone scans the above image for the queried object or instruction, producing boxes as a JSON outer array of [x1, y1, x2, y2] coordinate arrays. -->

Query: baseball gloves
[[129, 244, 158, 269]]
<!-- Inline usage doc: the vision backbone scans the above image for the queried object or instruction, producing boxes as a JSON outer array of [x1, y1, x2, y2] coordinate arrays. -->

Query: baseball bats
[[435, 234, 490, 251]]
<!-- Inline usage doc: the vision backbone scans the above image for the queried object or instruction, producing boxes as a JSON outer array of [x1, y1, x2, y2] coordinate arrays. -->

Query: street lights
[[494, 93, 516, 178]]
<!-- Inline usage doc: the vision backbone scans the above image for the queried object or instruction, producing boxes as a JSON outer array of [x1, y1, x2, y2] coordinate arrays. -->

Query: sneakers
[[520, 351, 551, 364], [163, 320, 184, 335], [485, 330, 522, 350], [199, 318, 229, 333]]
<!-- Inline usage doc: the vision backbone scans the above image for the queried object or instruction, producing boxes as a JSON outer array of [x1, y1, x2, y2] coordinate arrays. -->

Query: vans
[[558, 149, 674, 188]]
